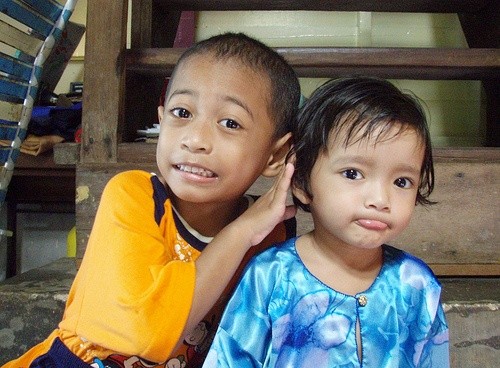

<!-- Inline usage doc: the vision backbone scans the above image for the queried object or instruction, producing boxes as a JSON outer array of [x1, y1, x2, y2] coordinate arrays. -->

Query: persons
[[0, 31, 302, 368], [184, 74, 454, 368]]
